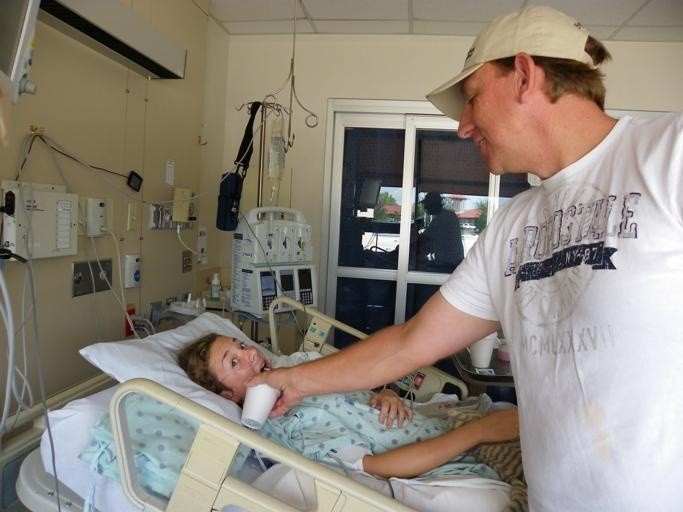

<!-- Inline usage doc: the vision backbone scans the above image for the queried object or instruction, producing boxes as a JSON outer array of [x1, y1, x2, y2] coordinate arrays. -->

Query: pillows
[[77, 311, 279, 424]]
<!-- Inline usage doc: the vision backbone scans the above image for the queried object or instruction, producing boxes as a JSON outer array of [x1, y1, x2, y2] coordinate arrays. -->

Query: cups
[[466, 336, 495, 368], [241, 381, 281, 431]]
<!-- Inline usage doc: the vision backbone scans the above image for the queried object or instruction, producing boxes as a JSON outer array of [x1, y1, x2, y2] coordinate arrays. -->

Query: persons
[[240, 4, 683, 512], [175, 333, 527, 483], [412, 189, 464, 271]]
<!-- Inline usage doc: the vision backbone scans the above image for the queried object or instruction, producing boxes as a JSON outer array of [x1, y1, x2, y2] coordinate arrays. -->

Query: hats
[[420, 192, 442, 203], [426, 6, 602, 121]]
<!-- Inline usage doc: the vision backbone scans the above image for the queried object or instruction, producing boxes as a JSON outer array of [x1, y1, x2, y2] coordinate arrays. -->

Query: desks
[[446, 335, 515, 398]]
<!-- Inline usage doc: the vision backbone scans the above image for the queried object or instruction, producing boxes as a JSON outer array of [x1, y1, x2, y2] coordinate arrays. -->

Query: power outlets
[[148, 203, 195, 231], [124, 302, 137, 337]]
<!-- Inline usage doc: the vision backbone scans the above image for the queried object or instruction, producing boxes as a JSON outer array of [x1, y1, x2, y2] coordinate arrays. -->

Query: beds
[[12, 293, 531, 512]]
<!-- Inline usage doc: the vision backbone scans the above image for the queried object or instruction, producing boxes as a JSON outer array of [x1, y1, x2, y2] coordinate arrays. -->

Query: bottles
[[211, 273, 220, 302]]
